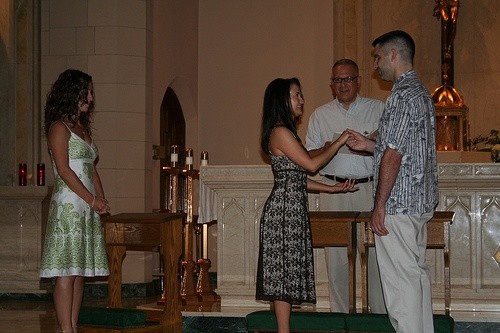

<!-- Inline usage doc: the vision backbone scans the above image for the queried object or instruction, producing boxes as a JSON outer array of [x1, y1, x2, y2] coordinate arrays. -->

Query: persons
[[346, 30, 440, 333], [39, 69, 111, 333], [304, 58, 388, 314], [261, 77, 360, 333]]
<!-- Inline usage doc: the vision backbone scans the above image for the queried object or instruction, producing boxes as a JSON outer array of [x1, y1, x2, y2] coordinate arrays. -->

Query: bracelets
[[90, 196, 96, 208]]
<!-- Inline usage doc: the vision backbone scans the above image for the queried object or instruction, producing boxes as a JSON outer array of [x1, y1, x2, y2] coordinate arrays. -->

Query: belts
[[324, 174, 374, 184]]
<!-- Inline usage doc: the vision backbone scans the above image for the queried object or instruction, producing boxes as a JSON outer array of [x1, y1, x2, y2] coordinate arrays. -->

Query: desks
[[101, 211, 186, 333], [269, 208, 455, 316]]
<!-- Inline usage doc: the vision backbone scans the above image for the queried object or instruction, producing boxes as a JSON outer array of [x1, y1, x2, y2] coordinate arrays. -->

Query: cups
[[37, 163, 45, 186], [19, 164, 27, 186]]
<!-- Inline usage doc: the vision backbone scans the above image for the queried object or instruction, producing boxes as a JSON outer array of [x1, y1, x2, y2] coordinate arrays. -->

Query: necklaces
[[71, 127, 87, 135]]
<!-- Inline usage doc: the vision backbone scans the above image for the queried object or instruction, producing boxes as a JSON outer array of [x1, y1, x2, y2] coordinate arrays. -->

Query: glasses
[[330, 75, 359, 83]]
